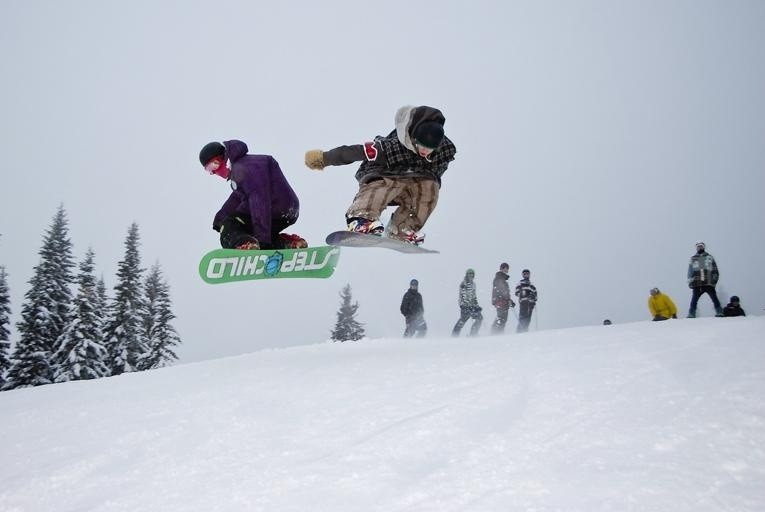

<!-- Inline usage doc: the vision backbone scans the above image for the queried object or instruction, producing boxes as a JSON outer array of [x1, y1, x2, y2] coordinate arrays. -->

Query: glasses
[[204, 155, 223, 176]]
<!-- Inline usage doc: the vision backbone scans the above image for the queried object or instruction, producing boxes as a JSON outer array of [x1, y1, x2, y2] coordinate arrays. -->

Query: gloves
[[306, 150, 325, 171]]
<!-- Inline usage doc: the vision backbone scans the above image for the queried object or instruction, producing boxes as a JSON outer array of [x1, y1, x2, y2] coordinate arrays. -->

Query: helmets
[[200, 142, 225, 166]]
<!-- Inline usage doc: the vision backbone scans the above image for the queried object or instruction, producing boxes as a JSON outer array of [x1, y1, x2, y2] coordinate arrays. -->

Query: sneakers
[[236, 239, 259, 249], [388, 225, 424, 246], [347, 219, 384, 235], [277, 233, 308, 248]]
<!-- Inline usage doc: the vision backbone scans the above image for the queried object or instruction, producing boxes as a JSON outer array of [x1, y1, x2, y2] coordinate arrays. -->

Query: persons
[[647, 287, 678, 321], [197, 138, 308, 251], [686, 240, 722, 318], [722, 295, 746, 317], [490, 262, 516, 335], [452, 268, 483, 336], [400, 278, 427, 339], [304, 104, 456, 244], [515, 270, 538, 333]]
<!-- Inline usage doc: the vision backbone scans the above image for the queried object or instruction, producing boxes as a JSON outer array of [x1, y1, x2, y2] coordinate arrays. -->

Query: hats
[[414, 122, 443, 148], [696, 242, 705, 249]]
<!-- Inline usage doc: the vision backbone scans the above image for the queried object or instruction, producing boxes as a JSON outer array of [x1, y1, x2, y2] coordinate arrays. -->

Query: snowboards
[[196, 246, 344, 284], [328, 232, 440, 257]]
